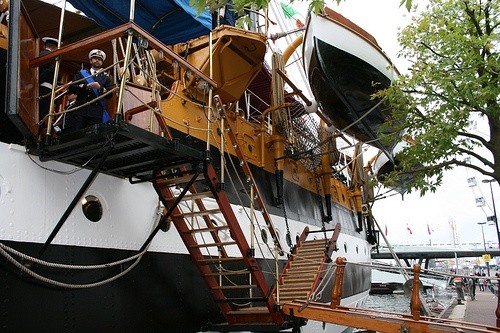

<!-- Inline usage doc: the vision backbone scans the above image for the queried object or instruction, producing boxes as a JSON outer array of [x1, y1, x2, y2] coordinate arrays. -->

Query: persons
[[67, 49, 110, 127], [452, 273, 486, 304]]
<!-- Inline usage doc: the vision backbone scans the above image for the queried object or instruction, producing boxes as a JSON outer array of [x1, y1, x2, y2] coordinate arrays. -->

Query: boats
[[372, 139, 426, 201], [0, 0, 381, 333], [300, 4, 409, 165]]
